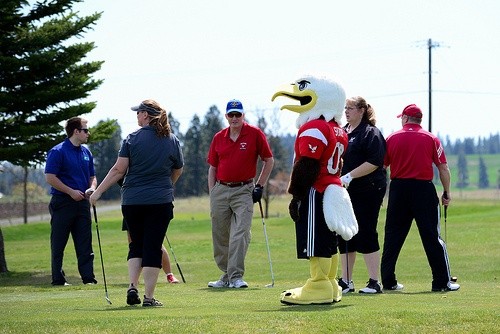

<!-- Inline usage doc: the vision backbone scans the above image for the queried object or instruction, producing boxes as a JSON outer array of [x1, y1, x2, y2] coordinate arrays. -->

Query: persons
[[89, 99, 184, 307], [44, 117, 97, 286], [384, 104, 460, 293], [338, 97, 387, 294], [206, 99, 274, 287]]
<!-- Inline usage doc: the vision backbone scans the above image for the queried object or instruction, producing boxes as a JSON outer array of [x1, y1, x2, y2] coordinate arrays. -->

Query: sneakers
[[392, 284, 403, 290], [208, 280, 229, 287], [432, 281, 461, 291], [359, 279, 383, 294], [229, 279, 248, 288], [338, 278, 355, 293]]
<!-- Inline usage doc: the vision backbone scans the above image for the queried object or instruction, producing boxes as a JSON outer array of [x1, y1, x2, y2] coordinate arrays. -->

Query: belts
[[217, 179, 253, 187]]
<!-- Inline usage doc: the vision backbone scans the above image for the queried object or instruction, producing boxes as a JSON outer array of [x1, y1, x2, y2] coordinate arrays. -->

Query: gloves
[[252, 184, 264, 203], [339, 173, 353, 189]]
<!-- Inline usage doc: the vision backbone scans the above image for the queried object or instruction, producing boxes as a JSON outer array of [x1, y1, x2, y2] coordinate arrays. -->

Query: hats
[[397, 104, 422, 118], [226, 99, 244, 114], [130, 104, 162, 116]]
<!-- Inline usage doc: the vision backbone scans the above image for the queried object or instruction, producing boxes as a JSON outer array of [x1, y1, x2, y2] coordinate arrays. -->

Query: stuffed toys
[[270, 76, 359, 305]]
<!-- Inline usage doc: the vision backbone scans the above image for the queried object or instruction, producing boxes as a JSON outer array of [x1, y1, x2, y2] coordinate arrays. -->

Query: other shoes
[[167, 273, 179, 283], [127, 286, 142, 305], [142, 295, 163, 306]]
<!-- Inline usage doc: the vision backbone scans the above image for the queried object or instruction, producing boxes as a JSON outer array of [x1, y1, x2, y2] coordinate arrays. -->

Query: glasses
[[345, 106, 360, 111], [228, 112, 242, 118], [78, 128, 88, 133], [137, 112, 140, 115]]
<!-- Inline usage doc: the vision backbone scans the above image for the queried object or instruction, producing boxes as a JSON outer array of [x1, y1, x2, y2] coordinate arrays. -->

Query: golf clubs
[[443, 190, 458, 282], [341, 241, 350, 295], [92, 203, 112, 306], [253, 178, 275, 289], [165, 234, 187, 284]]
